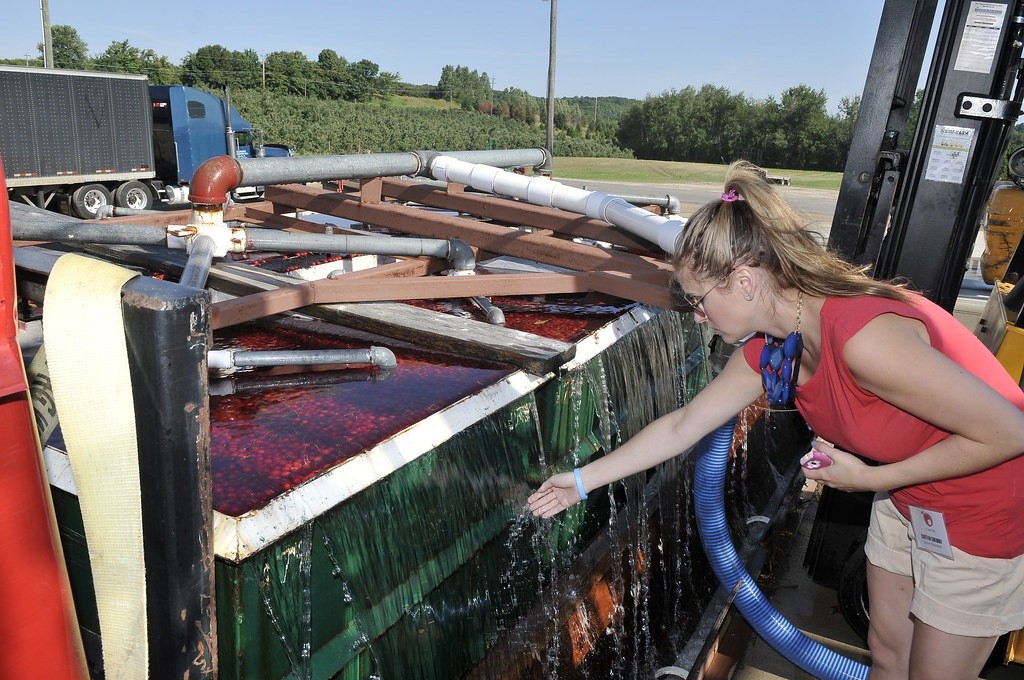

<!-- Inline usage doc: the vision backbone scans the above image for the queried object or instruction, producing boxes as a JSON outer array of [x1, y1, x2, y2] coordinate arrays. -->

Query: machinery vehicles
[[804, 0, 1023, 680]]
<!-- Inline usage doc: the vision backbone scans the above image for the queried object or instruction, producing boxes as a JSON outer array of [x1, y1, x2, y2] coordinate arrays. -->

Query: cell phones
[[800, 450, 834, 470]]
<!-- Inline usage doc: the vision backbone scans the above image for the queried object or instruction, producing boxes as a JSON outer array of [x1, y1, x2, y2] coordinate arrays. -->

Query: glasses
[[683, 263, 760, 318]]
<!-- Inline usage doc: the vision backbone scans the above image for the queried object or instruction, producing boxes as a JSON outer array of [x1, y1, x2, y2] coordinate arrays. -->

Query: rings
[[555, 499, 559, 504]]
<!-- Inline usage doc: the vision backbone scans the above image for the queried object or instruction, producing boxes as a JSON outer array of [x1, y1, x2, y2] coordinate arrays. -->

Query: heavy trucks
[[1, 63, 294, 223]]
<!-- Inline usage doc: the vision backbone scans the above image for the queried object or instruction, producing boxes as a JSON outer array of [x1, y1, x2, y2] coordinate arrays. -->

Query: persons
[[527, 158, 1024, 680]]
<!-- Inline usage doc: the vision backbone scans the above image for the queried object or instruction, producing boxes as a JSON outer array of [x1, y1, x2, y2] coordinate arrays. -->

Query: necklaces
[[757, 290, 804, 406]]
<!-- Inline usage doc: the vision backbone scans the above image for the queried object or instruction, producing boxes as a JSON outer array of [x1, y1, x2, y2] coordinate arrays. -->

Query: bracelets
[[573, 468, 588, 500]]
